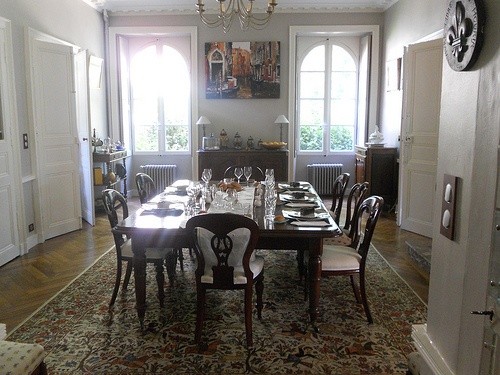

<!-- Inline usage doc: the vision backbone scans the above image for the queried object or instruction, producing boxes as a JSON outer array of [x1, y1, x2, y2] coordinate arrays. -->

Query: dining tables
[[111, 180, 344, 333]]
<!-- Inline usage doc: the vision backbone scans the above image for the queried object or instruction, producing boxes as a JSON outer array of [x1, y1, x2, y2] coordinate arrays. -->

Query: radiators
[[139, 165, 176, 196], [307, 163, 343, 196]]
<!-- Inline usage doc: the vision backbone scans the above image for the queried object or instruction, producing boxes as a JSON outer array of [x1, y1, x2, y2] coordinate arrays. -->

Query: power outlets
[[29, 223, 34, 232]]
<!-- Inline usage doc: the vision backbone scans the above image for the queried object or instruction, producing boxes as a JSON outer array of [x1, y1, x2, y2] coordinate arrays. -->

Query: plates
[[274, 219, 290, 223], [365, 142, 387, 148], [278, 182, 329, 220]]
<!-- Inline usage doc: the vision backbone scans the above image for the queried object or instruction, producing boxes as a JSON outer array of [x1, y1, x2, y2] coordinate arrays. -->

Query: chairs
[[101, 173, 265, 346], [298, 172, 384, 324]]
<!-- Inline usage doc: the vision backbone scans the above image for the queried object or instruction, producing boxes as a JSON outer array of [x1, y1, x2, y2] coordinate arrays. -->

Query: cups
[[264, 169, 277, 230]]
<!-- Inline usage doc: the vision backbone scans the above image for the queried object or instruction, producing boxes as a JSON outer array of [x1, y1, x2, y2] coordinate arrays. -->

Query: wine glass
[[186, 181, 200, 216], [201, 169, 212, 188], [225, 188, 236, 212], [244, 167, 252, 188], [234, 168, 243, 184]]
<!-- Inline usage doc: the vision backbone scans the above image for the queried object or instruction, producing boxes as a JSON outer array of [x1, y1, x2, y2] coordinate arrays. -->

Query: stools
[[0, 340, 45, 375]]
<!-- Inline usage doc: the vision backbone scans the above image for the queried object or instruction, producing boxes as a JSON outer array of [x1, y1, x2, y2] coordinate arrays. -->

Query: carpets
[[3, 225, 429, 375]]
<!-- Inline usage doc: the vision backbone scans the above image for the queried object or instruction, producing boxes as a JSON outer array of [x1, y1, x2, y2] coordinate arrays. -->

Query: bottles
[[220, 129, 227, 150], [258, 138, 263, 150], [370, 126, 383, 144], [233, 132, 241, 150], [247, 136, 254, 151]]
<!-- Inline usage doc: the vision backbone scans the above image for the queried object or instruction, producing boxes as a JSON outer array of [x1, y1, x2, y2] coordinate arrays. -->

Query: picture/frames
[[386, 59, 400, 92], [89, 55, 104, 89]]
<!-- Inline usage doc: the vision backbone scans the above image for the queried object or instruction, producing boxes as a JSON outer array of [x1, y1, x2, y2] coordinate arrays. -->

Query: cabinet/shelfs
[[93, 151, 128, 212], [355, 145, 398, 213], [197, 150, 289, 182]]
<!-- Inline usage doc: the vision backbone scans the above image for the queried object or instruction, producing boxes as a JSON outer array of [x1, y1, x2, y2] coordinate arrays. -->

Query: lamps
[[196, 116, 212, 137], [273, 115, 290, 142], [195, 0, 278, 33]]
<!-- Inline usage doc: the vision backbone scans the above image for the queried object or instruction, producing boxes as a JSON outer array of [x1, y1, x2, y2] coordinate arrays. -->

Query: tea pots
[[103, 137, 114, 146]]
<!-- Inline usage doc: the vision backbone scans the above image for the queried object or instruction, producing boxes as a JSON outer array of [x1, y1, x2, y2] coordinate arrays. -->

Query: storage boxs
[[94, 168, 103, 185]]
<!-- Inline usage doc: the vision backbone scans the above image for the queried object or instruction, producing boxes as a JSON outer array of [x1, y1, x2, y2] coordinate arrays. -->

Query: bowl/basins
[[261, 144, 286, 149]]
